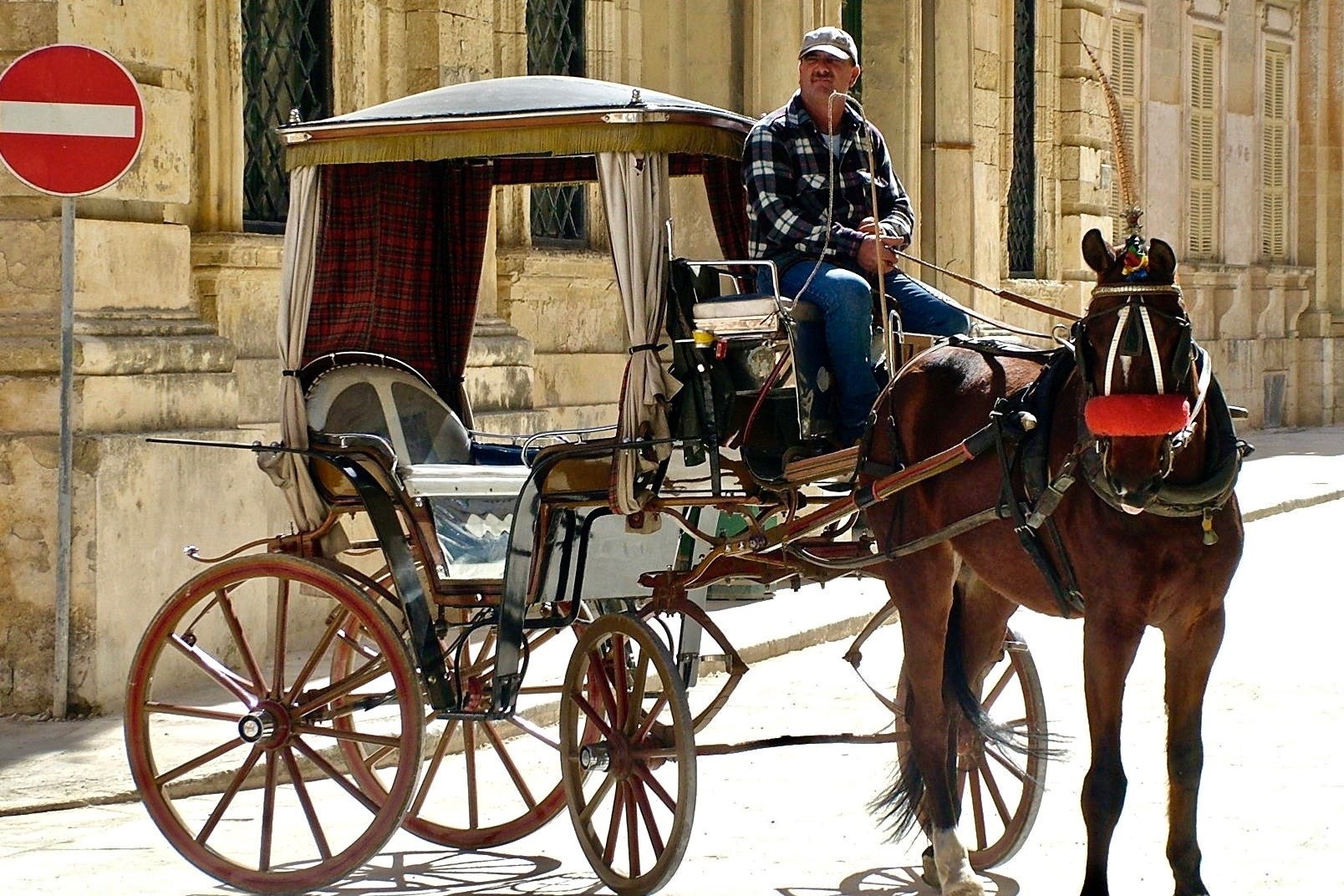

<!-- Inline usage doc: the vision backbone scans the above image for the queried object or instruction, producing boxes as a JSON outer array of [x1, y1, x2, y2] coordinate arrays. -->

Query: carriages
[[115, 75, 1249, 896]]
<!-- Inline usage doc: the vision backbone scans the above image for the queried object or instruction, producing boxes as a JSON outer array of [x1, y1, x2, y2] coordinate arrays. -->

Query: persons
[[742, 26, 972, 451]]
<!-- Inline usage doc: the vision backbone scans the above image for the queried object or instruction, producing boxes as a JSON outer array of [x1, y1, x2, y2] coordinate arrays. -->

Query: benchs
[[307, 366, 561, 486]]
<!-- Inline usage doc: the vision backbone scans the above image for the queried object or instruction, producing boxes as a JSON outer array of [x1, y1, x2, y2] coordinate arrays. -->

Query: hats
[[798, 26, 858, 67]]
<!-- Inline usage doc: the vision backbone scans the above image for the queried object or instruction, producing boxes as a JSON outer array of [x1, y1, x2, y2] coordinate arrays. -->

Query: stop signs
[[0, 44, 148, 195]]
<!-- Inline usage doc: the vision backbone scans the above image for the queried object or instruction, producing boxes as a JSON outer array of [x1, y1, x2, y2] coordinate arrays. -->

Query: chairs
[[678, 259, 896, 337]]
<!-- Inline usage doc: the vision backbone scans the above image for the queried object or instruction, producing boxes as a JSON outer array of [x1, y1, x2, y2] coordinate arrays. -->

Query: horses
[[856, 229, 1246, 896]]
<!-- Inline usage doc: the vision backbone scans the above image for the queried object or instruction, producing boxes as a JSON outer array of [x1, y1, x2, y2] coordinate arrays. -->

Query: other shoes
[[843, 424, 866, 449]]
